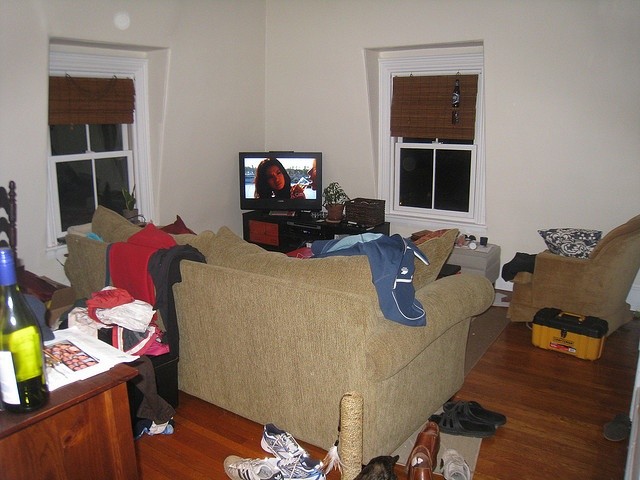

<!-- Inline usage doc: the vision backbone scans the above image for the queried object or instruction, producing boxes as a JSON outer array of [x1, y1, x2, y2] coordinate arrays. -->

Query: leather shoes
[[408, 445, 432, 480], [405, 421, 440, 474]]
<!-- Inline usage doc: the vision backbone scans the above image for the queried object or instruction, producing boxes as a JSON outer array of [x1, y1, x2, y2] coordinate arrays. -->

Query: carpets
[[387, 402, 485, 480]]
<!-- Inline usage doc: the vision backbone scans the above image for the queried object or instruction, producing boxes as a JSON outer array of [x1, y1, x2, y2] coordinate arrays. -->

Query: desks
[[241, 210, 391, 256], [0, 266, 143, 478]]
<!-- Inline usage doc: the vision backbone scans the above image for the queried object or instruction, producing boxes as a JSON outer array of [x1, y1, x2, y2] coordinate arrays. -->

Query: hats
[[22, 294, 54, 341]]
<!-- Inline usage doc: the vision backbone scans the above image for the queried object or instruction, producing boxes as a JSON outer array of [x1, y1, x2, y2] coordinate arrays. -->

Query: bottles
[[1, 245, 49, 414]]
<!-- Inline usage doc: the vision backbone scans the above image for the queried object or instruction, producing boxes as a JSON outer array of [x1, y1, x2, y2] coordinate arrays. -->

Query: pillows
[[410, 227, 460, 293], [204, 225, 405, 300], [91, 204, 214, 264], [538, 228, 604, 260]]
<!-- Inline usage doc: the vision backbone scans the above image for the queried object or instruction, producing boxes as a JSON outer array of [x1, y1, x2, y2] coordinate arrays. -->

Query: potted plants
[[322, 176, 350, 222]]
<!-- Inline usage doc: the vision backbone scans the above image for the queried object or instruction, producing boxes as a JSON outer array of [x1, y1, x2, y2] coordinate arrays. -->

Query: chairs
[[0, 180, 18, 263]]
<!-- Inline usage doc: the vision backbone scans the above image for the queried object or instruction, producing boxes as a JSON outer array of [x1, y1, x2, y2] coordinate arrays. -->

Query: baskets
[[345, 197, 385, 226]]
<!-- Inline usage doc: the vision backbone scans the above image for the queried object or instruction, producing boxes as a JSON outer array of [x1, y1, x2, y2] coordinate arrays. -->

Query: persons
[[253, 158, 292, 199], [290, 159, 316, 200]]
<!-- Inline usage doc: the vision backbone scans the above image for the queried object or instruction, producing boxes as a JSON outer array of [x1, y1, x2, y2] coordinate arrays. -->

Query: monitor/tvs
[[238, 151, 323, 217]]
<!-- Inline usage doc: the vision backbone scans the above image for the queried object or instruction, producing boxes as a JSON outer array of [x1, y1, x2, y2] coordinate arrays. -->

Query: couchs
[[501, 214, 640, 339], [62, 203, 495, 469]]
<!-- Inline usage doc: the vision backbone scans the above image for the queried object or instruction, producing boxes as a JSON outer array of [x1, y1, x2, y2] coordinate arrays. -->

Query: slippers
[[443, 400, 506, 426], [428, 410, 495, 438]]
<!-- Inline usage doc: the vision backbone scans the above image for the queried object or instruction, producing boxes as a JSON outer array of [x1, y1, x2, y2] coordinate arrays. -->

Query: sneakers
[[261, 424, 308, 459], [261, 457, 326, 480], [223, 455, 284, 480], [603, 414, 632, 441], [440, 448, 471, 480]]
